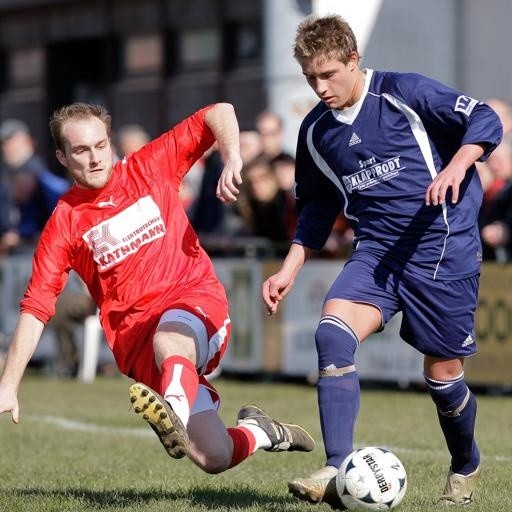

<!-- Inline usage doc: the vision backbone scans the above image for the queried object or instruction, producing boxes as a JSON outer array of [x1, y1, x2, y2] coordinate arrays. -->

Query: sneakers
[[236, 404, 315, 452], [434, 464, 481, 509], [287, 465, 347, 512], [128, 382, 189, 459]]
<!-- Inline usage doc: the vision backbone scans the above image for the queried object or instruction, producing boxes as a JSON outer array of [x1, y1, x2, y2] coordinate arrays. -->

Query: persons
[[473, 99, 512, 264], [260, 11, 504, 504], [0, 119, 72, 256], [43, 272, 104, 383], [111, 113, 356, 260], [0, 99, 318, 475]]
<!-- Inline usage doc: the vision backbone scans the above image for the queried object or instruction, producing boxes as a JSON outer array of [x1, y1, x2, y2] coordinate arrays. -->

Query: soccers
[[335, 447, 407, 512]]
